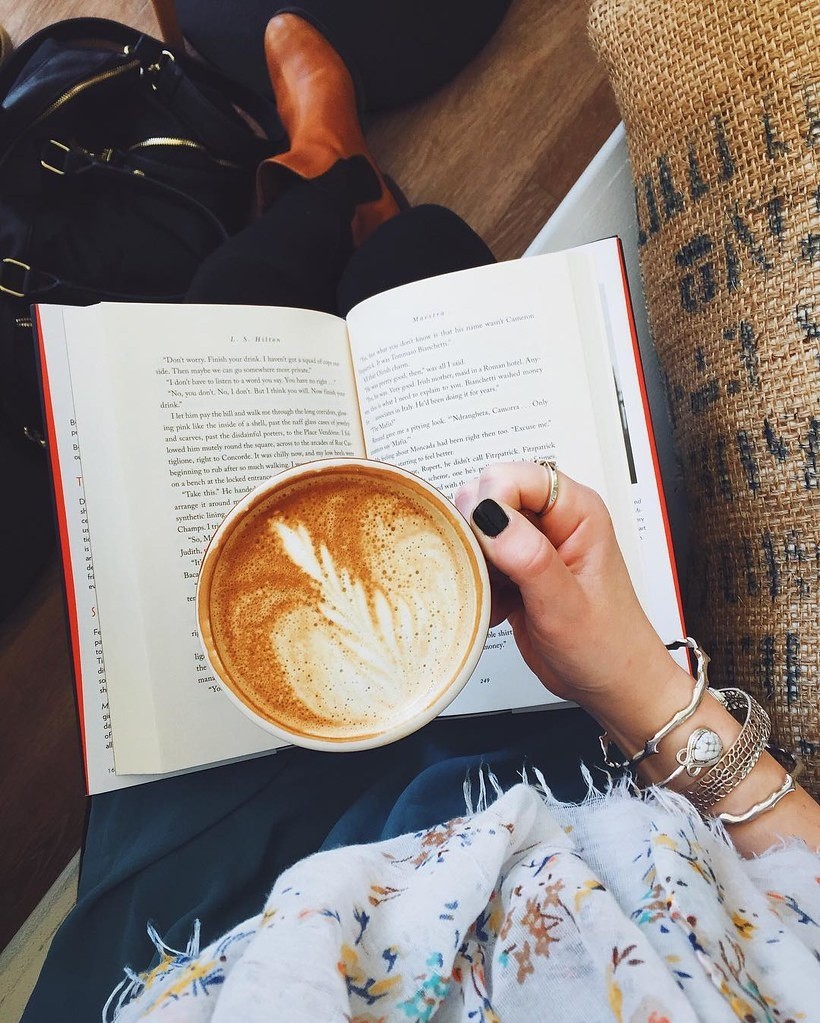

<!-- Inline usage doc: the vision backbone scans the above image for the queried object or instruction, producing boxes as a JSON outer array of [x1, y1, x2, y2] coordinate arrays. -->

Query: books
[[27, 235, 694, 800]]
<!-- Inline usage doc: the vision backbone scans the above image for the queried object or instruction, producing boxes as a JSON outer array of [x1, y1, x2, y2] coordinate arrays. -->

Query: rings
[[537, 459, 558, 518]]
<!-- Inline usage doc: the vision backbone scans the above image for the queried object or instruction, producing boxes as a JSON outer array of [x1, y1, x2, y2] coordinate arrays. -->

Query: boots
[[254, 6, 411, 244]]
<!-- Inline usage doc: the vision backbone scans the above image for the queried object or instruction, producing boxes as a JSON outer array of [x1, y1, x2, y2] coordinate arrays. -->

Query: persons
[[0, 10, 819, 1023]]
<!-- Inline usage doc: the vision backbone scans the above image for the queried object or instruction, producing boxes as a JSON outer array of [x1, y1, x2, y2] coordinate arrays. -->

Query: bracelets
[[703, 747, 803, 830], [637, 684, 771, 814], [598, 636, 710, 768]]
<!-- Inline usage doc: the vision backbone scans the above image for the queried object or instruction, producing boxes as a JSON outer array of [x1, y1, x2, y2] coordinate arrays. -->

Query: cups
[[195, 457, 500, 753]]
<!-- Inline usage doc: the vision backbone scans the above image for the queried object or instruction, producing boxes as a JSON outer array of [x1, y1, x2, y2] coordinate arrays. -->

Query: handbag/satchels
[[0, 38, 288, 448]]
